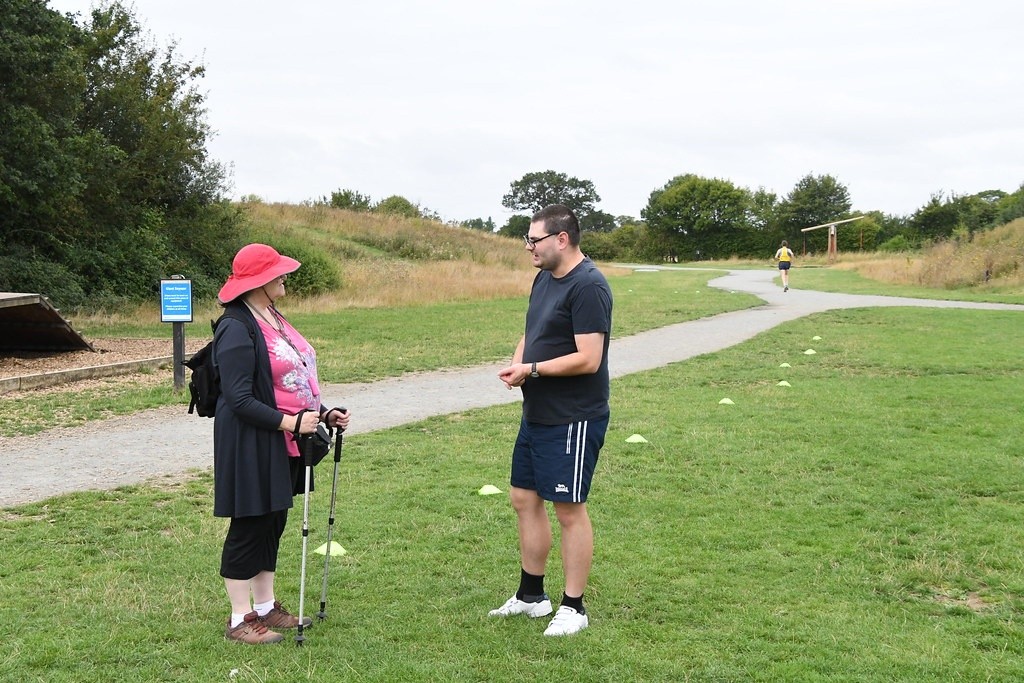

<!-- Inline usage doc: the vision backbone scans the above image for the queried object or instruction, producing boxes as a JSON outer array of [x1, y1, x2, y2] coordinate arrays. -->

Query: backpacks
[[181, 314, 256, 419]]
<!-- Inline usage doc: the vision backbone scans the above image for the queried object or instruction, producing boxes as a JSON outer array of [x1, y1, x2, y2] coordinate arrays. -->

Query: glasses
[[523, 230, 560, 249]]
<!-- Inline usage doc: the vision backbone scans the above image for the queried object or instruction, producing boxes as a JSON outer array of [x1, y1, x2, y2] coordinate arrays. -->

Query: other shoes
[[784, 286, 788, 292]]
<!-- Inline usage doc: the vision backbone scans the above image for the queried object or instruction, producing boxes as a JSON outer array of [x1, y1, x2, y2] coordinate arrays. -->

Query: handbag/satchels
[[298, 425, 336, 465]]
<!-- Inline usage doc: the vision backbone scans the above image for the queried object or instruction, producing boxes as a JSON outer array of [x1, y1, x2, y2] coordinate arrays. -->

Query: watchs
[[530, 362, 540, 378]]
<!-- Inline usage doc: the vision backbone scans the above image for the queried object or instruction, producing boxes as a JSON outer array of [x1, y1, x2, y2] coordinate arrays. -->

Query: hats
[[218, 243, 302, 303]]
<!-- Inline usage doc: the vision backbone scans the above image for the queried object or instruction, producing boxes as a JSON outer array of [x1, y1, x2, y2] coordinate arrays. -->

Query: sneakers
[[226, 610, 285, 644], [257, 600, 312, 630], [544, 604, 588, 636], [488, 589, 553, 618]]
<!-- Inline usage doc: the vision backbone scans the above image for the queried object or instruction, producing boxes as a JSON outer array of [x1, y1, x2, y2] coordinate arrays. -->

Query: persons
[[774, 240, 794, 292], [489, 204, 613, 637], [212, 243, 352, 644]]
